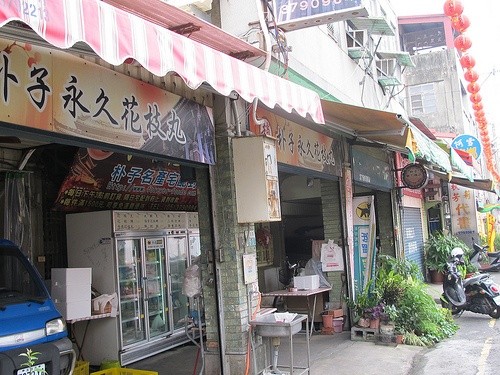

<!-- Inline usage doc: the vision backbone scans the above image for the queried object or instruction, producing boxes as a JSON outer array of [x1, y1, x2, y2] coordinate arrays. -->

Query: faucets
[[253, 285, 260, 294]]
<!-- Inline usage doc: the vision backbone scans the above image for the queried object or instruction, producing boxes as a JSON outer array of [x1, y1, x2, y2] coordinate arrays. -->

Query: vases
[[371, 320, 379, 329]]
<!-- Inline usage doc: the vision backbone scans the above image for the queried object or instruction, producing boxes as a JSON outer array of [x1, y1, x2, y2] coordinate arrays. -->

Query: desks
[[66, 314, 117, 362], [261, 289, 332, 340]]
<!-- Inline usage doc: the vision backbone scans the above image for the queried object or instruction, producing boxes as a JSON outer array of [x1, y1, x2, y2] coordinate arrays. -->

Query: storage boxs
[[50, 267, 91, 320], [294, 275, 321, 289], [287, 291, 329, 322], [329, 309, 343, 317], [92, 303, 111, 314], [90, 368, 158, 375], [72, 360, 89, 375]]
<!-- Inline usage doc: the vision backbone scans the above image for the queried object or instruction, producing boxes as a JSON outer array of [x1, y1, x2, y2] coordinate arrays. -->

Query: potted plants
[[394, 327, 404, 344], [343, 277, 376, 326], [424, 229, 475, 282]]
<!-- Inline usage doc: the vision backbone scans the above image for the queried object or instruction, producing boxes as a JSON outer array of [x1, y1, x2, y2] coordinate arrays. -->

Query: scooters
[[469, 235, 500, 273], [441, 252, 500, 318]]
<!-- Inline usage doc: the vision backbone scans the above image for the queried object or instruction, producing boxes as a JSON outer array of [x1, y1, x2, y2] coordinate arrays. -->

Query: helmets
[[451, 247, 464, 257]]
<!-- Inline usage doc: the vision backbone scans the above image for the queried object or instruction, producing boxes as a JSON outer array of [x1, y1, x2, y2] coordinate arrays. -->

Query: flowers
[[369, 302, 388, 321]]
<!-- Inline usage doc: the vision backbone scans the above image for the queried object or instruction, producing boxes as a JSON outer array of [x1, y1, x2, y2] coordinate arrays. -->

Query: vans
[[0, 239, 76, 375]]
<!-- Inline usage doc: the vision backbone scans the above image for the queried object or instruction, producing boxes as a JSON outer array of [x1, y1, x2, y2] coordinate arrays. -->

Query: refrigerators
[[65, 212, 205, 367]]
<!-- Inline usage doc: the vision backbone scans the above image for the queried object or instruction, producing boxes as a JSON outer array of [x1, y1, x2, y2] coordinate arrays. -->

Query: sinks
[[248, 312, 308, 338]]
